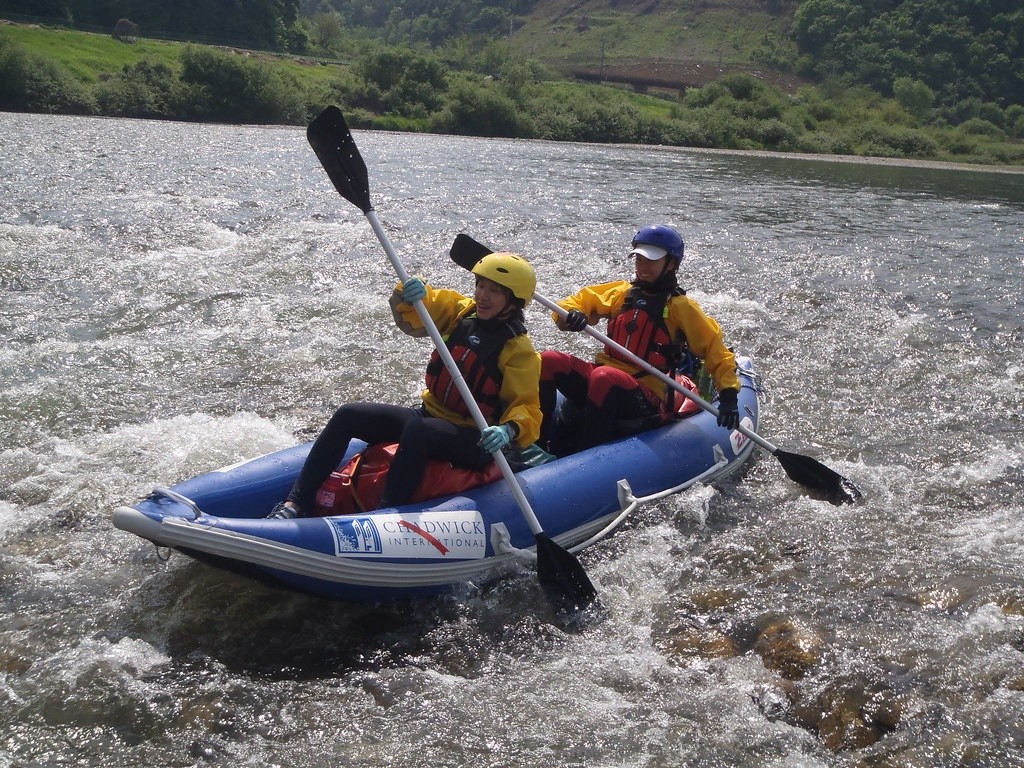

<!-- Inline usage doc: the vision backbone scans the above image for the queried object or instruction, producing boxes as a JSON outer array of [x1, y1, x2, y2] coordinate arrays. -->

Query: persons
[[534, 223, 741, 459], [256, 250, 544, 521]]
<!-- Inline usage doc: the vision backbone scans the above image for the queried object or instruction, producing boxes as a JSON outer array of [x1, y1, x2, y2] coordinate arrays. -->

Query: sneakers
[[262, 500, 306, 519]]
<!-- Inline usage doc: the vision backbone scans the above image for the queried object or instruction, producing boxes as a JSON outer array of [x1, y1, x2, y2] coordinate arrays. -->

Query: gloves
[[716, 388, 740, 430], [565, 309, 588, 333], [401, 274, 427, 304], [477, 424, 516, 453]]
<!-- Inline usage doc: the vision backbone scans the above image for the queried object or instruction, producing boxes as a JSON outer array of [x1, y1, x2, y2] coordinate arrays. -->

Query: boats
[[109, 353, 771, 597]]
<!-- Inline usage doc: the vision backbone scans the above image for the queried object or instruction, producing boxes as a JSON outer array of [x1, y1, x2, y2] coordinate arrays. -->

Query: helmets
[[470, 252, 536, 309], [631, 224, 683, 264]]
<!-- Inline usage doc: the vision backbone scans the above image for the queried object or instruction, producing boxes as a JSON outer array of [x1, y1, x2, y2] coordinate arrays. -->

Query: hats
[[628, 243, 669, 261]]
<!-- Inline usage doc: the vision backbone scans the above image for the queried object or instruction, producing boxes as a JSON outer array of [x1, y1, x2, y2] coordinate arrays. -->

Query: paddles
[[448, 232, 863, 506], [304, 103, 609, 636]]
